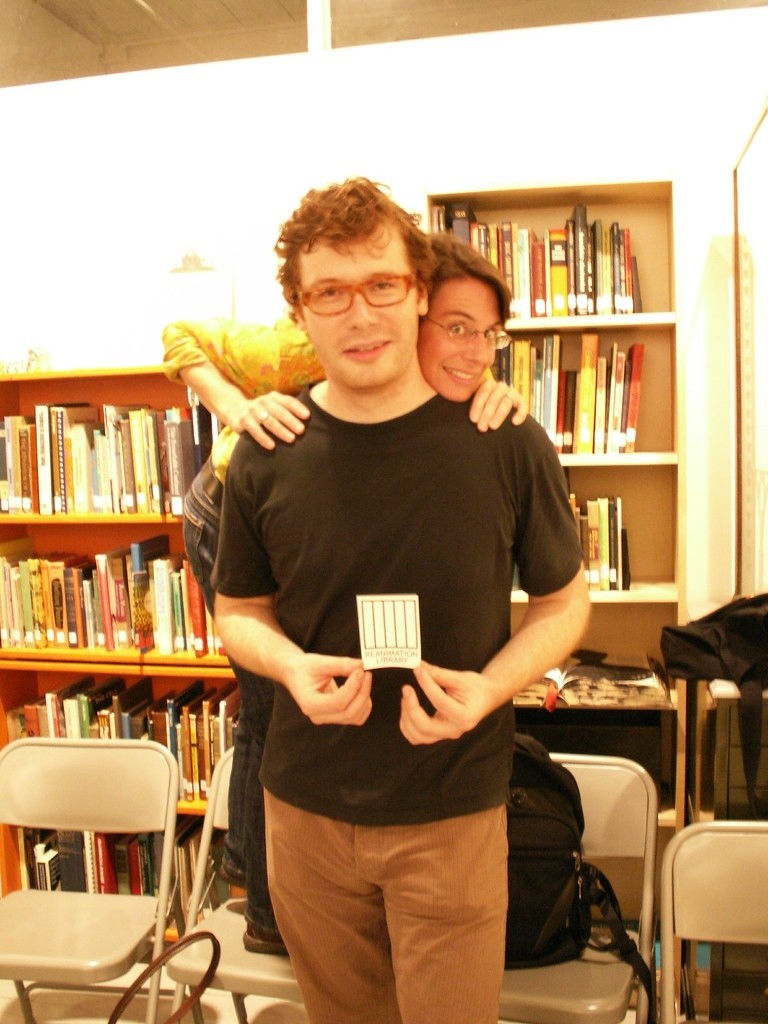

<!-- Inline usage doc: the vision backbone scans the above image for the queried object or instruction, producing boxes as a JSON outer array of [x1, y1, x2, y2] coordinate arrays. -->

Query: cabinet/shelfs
[[427, 179, 687, 921], [0, 368, 248, 941]]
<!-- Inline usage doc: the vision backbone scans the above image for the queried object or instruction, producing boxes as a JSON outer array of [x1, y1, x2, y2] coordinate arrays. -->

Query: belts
[[202, 455, 225, 508]]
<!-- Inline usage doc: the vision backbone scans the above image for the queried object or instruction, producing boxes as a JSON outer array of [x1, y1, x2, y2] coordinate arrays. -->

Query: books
[[435, 200, 646, 595], [0, 385, 241, 931]]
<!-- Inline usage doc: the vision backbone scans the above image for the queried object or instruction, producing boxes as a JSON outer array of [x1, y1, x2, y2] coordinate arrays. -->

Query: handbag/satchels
[[664, 592, 766, 820]]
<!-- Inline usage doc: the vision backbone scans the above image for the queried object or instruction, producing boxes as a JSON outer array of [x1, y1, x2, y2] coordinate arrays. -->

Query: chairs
[[658, 820, 768, 1024], [164, 747, 309, 1024], [0, 737, 205, 1024], [495, 751, 659, 1024]]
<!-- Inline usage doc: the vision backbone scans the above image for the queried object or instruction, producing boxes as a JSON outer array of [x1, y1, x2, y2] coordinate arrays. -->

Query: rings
[[259, 411, 268, 423], [506, 395, 514, 407]]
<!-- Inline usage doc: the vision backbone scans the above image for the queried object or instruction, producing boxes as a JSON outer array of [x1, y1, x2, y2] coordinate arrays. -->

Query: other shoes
[[218, 844, 248, 890], [243, 921, 291, 955]]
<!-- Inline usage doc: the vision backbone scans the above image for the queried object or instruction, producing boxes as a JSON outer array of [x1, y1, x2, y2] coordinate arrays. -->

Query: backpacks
[[506, 733, 663, 1023]]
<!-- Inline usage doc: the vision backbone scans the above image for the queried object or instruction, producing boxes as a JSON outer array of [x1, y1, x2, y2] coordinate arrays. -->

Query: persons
[[211, 179, 592, 1024], [163, 229, 531, 954]]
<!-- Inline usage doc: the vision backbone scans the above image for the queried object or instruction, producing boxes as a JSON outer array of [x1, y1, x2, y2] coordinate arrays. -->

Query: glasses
[[293, 273, 419, 316], [425, 316, 511, 351]]
